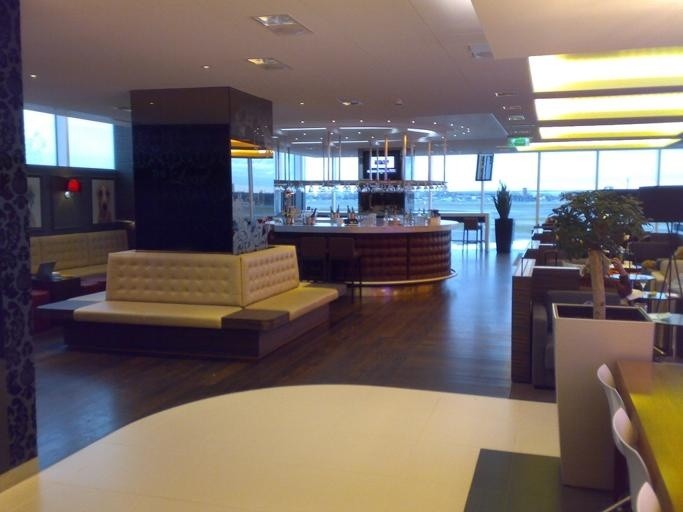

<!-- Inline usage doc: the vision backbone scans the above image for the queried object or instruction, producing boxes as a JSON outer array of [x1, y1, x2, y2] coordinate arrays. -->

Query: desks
[[608, 263, 682, 362], [439, 212, 489, 253]]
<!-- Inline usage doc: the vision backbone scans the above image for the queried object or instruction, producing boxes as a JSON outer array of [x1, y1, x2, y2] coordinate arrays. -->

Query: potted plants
[[531, 186, 655, 491], [490, 179, 513, 253]]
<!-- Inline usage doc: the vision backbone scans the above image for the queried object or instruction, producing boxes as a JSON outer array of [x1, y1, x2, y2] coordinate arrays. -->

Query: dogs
[[96, 184, 112, 223]]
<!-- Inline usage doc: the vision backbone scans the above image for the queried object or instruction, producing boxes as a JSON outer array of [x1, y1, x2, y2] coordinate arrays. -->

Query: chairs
[[462, 217, 481, 244], [530, 290, 619, 390], [595, 362, 660, 512]]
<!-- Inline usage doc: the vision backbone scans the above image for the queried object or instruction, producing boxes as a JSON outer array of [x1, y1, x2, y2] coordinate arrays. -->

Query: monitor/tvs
[[365, 154, 397, 178]]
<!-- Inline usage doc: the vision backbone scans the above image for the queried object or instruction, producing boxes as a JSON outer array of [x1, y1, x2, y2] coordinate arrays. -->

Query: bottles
[[302, 204, 355, 224]]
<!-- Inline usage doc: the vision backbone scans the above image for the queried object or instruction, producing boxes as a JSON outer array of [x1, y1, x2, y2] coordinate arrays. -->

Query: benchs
[[36, 244, 346, 363]]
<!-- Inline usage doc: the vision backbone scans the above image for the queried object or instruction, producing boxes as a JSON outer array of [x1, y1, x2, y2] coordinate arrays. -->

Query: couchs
[[27, 220, 136, 334]]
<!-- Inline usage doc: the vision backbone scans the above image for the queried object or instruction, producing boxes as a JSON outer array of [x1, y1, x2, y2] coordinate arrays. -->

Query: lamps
[[639, 185, 682, 313]]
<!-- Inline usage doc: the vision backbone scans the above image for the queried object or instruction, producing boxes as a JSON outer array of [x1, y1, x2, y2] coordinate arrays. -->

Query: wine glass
[[276, 182, 446, 192]]
